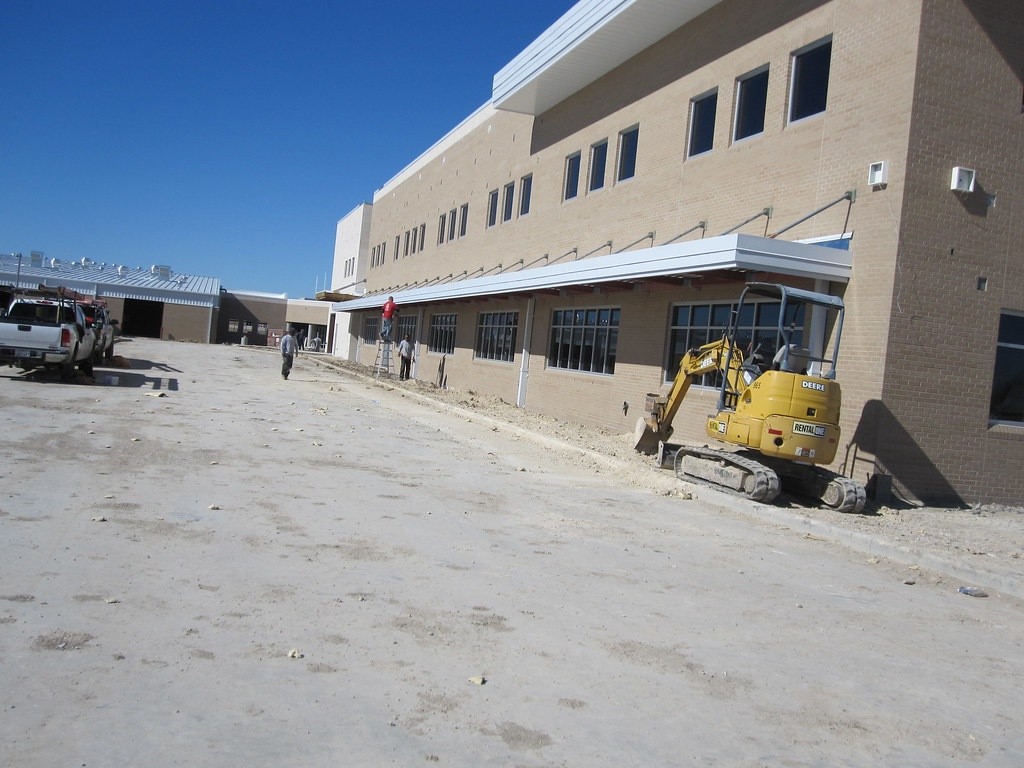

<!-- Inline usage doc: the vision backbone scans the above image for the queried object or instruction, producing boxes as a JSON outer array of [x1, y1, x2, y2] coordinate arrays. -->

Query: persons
[[297, 329, 306, 351], [378, 296, 401, 343], [397, 334, 416, 381], [280, 328, 299, 380]]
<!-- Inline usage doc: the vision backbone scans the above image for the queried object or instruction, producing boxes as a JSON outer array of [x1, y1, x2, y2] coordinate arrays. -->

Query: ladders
[[372, 340, 395, 377]]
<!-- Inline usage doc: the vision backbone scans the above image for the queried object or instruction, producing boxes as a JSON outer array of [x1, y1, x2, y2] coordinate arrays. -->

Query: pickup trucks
[[0, 296, 95, 380], [78, 302, 117, 362]]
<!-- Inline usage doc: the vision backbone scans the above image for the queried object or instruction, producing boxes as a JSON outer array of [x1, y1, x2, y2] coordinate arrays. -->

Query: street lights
[[14, 253, 23, 297]]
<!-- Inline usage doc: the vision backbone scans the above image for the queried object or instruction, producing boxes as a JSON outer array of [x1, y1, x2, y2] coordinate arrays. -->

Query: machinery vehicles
[[630, 280, 867, 516]]
